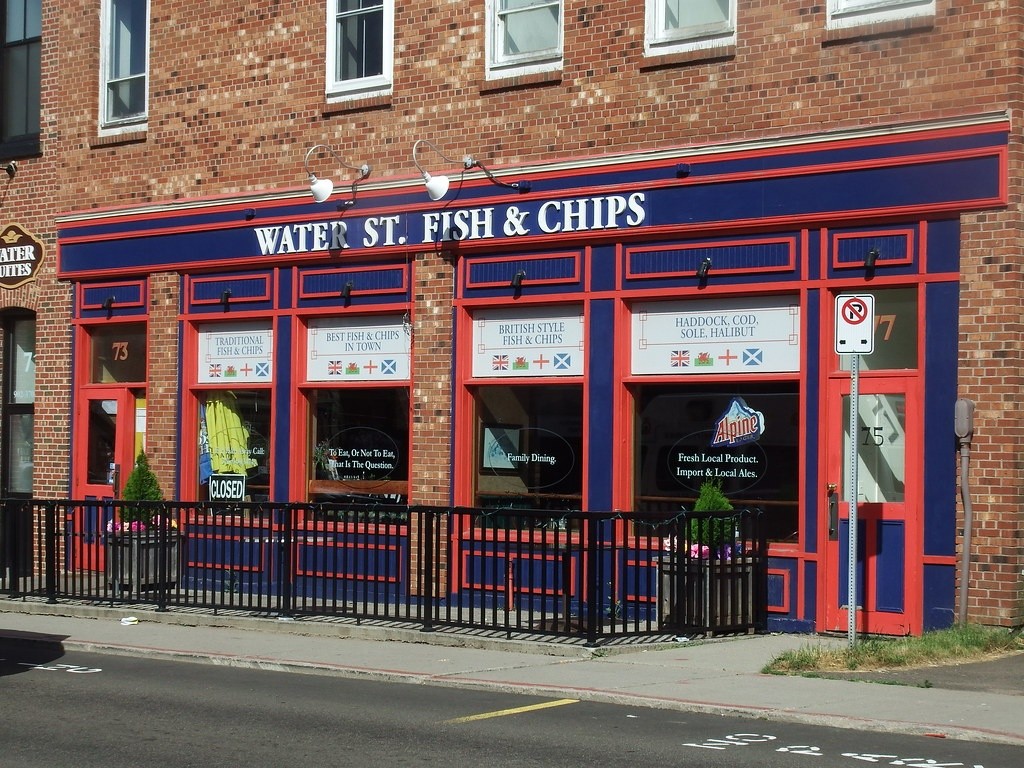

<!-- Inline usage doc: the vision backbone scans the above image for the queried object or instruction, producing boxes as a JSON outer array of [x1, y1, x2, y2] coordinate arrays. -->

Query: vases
[[103, 532, 185, 593], [653, 556, 759, 638]]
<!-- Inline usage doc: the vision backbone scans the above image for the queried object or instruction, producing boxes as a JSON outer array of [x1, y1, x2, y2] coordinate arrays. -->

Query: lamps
[[413, 140, 475, 201], [305, 145, 370, 203]]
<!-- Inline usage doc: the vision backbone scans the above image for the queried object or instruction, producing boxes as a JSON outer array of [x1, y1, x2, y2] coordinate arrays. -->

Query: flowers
[[106, 449, 178, 532], [664, 477, 750, 560]]
